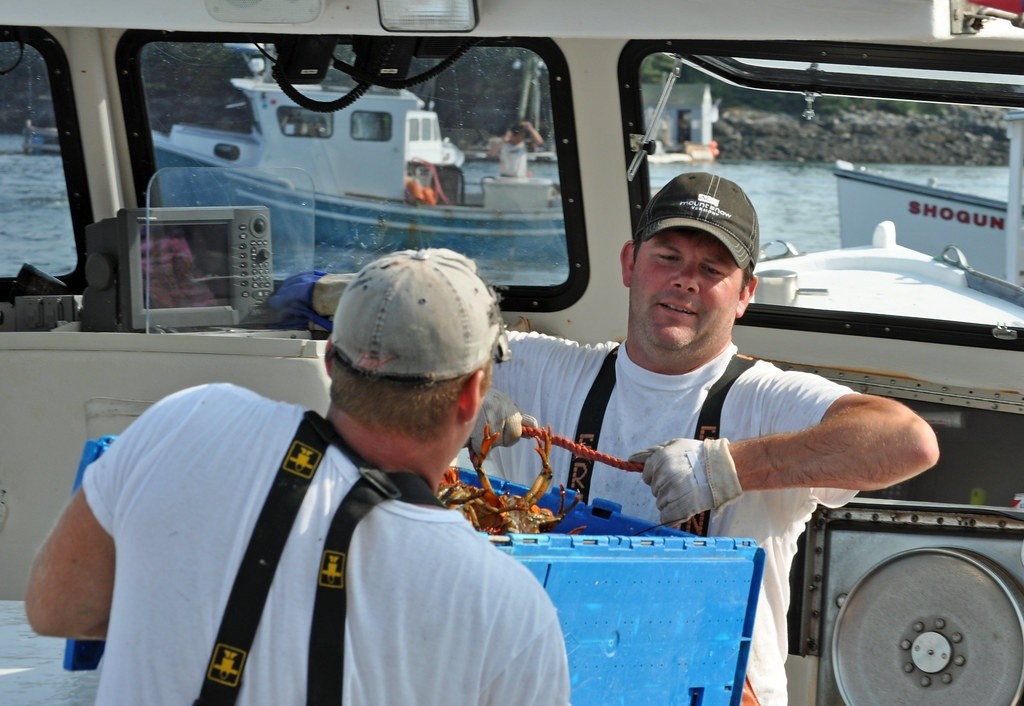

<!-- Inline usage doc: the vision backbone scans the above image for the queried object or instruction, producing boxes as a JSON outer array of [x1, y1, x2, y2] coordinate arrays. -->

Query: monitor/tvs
[[139, 222, 232, 308]]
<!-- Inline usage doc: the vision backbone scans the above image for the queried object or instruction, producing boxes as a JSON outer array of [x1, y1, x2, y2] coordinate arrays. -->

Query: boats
[[151, 44, 571, 284], [836, 160, 1024, 289]]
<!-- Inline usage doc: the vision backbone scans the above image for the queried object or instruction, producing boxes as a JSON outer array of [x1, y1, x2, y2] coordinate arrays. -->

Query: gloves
[[629, 437, 743, 526], [470, 387, 538, 455]]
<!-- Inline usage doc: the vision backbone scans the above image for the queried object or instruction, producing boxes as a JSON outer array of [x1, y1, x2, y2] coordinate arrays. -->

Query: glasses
[[486, 333, 512, 363]]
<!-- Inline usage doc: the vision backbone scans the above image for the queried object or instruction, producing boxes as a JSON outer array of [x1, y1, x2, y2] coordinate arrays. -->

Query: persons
[[467, 172, 941, 706], [23, 245, 573, 706]]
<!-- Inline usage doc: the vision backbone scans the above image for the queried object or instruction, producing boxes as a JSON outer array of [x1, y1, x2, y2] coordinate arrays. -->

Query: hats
[[332, 247, 505, 381], [636, 172, 759, 274]]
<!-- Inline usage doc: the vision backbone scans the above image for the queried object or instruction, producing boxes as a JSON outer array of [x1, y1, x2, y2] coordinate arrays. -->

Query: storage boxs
[[60, 435, 768, 706]]
